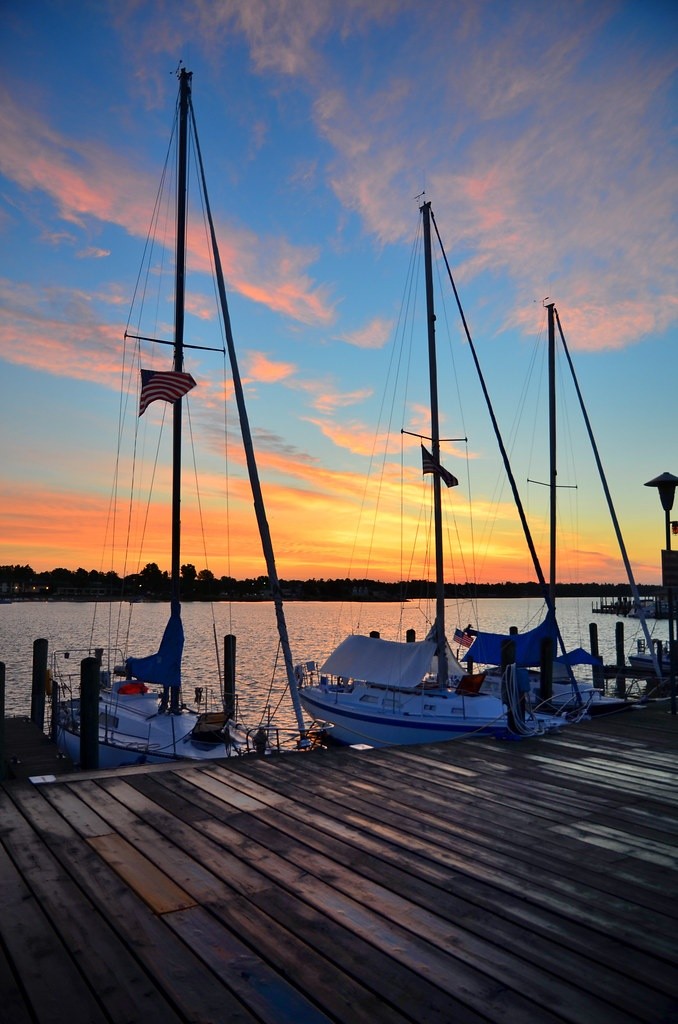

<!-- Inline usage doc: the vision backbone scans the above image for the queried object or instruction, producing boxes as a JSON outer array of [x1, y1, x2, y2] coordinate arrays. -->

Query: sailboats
[[296, 199, 584, 747], [459, 302, 670, 719], [50, 66, 312, 771]]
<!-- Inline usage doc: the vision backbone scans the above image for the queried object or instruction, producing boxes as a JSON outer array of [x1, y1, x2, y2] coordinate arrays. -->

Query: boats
[[628, 640, 670, 671], [625, 608, 651, 618]]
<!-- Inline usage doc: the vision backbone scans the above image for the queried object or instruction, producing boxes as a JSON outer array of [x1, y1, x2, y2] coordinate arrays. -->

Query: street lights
[[644, 472, 678, 714]]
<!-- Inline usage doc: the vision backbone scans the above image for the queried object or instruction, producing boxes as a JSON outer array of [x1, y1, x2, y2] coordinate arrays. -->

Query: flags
[[422, 445, 458, 488], [139, 369, 197, 417], [453, 629, 473, 648]]
